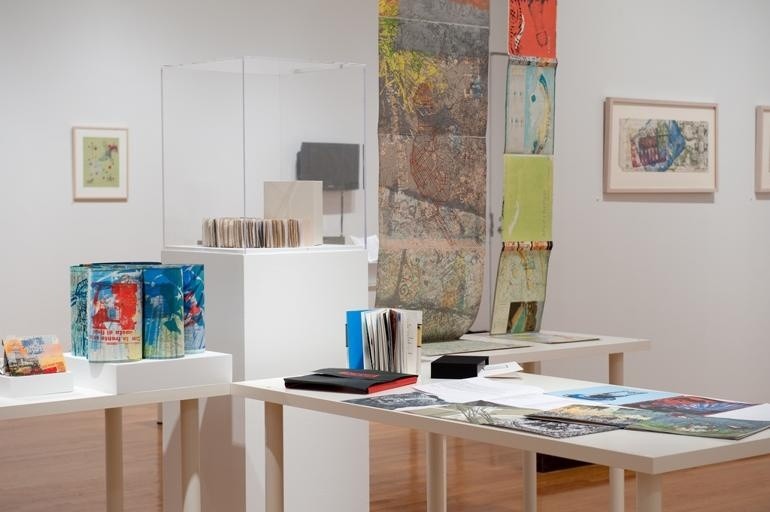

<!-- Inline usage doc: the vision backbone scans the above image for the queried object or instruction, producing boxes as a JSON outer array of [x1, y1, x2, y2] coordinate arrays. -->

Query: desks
[[420, 329, 652, 512], [230, 359, 770, 512], [0, 345, 232, 512]]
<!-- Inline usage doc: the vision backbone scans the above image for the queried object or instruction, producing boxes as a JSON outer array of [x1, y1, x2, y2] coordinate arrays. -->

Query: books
[[346, 306, 423, 380], [282, 365, 419, 395]]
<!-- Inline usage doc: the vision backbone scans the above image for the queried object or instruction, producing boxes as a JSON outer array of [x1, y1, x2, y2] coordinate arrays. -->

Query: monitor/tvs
[[297, 142, 360, 189]]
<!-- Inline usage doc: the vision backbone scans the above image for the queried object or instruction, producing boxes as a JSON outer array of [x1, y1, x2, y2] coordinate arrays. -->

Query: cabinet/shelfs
[[161, 52, 371, 512]]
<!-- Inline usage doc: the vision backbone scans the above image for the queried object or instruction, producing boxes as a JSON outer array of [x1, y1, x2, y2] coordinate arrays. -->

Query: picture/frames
[[72, 126, 129, 201], [755, 105, 770, 193], [603, 96, 718, 194]]
